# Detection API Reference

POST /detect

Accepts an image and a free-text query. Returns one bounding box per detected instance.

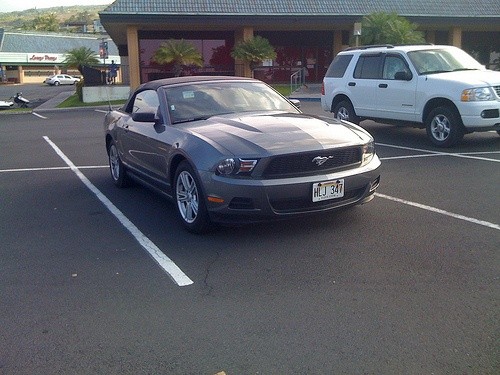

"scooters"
[0,91,34,110]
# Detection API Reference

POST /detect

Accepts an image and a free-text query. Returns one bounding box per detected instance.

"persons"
[107,60,119,85]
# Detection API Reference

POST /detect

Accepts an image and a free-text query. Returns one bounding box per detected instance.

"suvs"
[320,43,500,148]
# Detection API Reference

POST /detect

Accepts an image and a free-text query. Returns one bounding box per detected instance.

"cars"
[45,73,81,87]
[103,76,382,235]
